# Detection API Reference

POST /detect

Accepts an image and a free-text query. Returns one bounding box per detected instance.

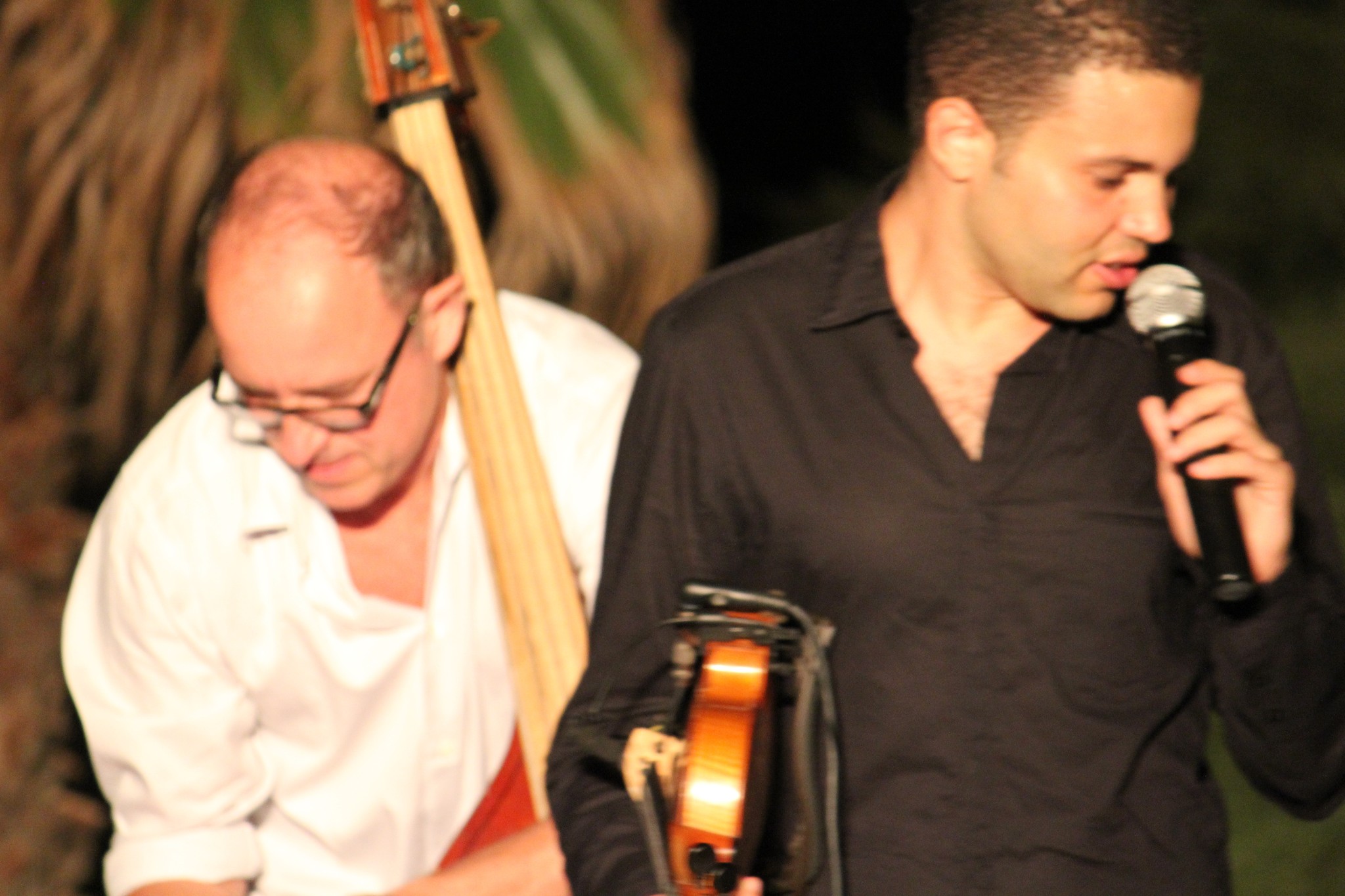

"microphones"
[1123,262,1253,604]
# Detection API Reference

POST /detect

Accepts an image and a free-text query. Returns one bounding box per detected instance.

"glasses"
[210,301,416,434]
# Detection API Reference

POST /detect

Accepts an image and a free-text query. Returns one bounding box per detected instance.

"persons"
[57,134,649,896]
[531,0,1345,896]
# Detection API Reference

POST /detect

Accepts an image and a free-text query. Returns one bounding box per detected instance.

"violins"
[617,580,789,896]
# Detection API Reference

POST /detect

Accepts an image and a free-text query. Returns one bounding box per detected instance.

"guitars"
[348,0,593,879]
